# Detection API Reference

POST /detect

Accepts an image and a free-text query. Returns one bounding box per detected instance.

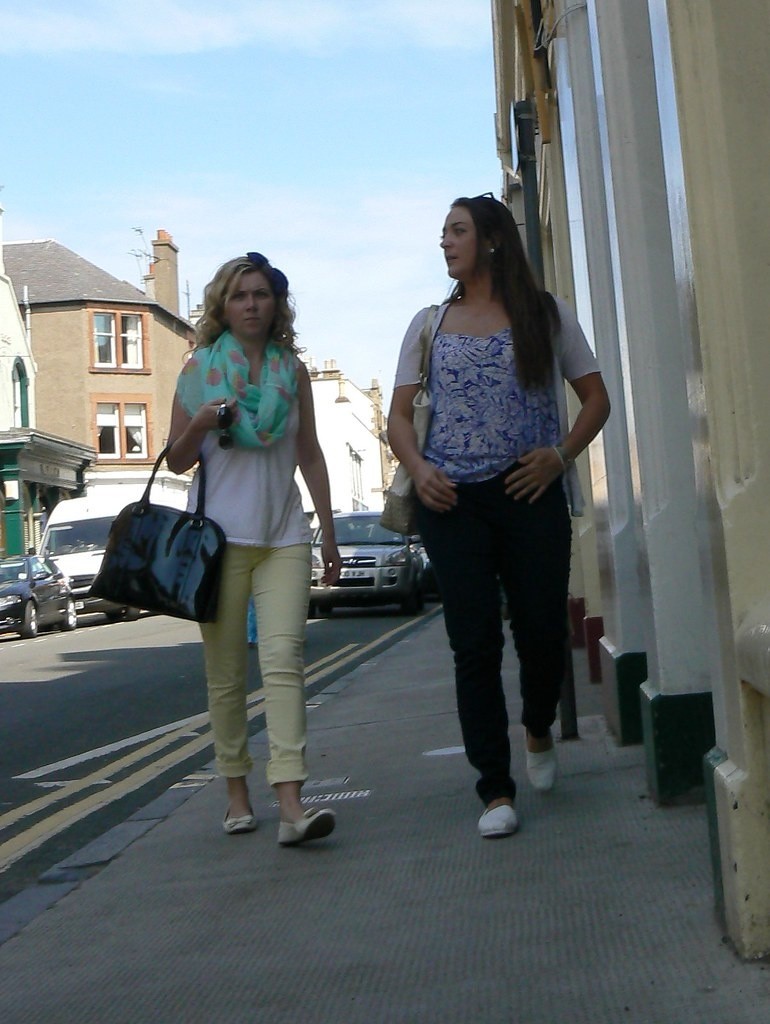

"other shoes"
[222,804,257,833]
[478,804,518,837]
[277,807,335,844]
[525,727,558,791]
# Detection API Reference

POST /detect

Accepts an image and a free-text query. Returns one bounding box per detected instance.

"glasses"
[217,404,234,451]
[473,192,497,204]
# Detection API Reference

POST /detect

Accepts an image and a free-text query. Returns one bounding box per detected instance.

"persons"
[164,251,342,841]
[388,192,612,837]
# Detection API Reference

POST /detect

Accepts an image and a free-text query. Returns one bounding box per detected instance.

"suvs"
[308,510,425,619]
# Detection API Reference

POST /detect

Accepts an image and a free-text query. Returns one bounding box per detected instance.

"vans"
[29,490,189,623]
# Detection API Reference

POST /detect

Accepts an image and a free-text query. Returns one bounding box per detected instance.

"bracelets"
[554,446,574,471]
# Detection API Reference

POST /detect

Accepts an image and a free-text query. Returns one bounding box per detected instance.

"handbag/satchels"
[87,445,226,624]
[379,303,451,536]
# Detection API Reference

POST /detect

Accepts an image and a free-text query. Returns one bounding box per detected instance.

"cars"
[415,540,440,598]
[1,555,77,638]
